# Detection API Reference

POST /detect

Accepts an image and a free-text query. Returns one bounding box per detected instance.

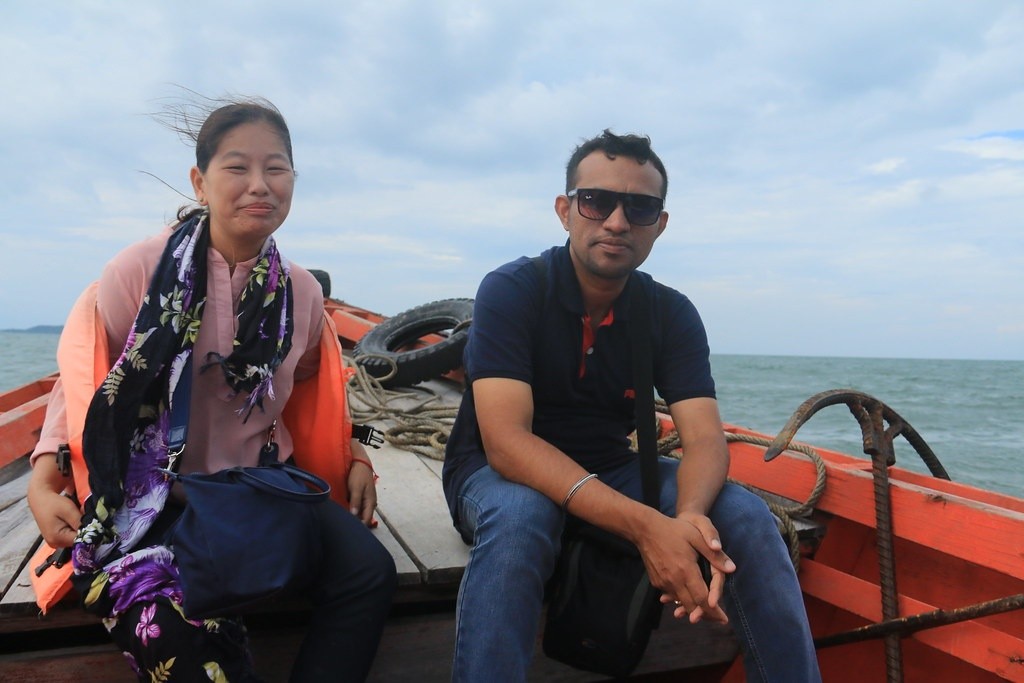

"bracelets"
[562,472,599,512]
[350,457,379,484]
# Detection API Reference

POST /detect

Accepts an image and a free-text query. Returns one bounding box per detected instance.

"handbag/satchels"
[543,533,663,678]
[150,460,398,625]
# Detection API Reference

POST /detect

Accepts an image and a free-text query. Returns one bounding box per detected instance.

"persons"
[29,98,397,683]
[439,132,820,683]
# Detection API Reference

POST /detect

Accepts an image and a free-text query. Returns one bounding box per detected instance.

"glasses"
[567,188,667,226]
